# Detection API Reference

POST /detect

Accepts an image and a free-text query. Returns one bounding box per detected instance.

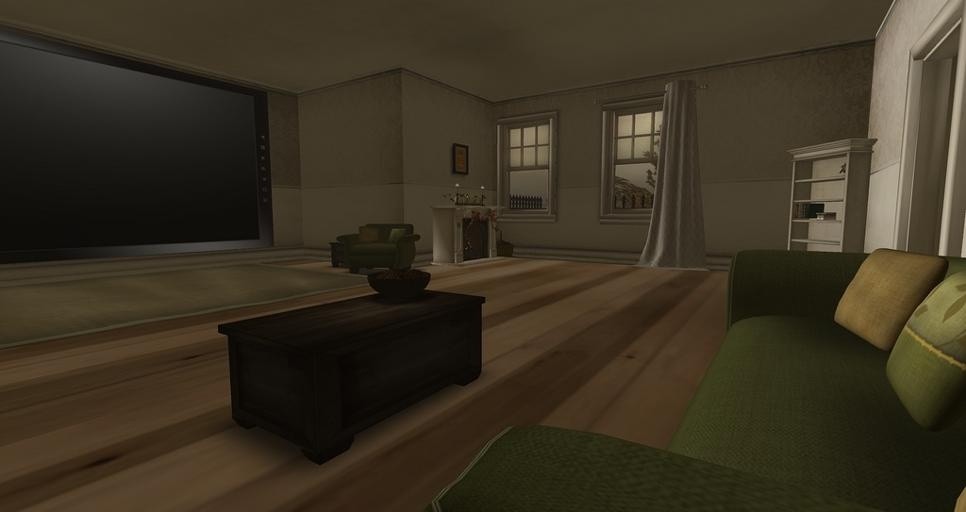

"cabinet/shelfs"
[785,137,875,255]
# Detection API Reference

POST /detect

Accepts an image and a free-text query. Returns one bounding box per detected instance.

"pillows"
[833,243,966,429]
[386,228,407,242]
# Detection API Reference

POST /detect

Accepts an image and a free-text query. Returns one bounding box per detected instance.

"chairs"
[337,222,419,272]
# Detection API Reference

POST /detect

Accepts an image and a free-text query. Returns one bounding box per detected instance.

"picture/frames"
[452,143,469,176]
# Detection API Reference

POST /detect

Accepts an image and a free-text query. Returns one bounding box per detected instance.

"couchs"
[428,250,966,512]
